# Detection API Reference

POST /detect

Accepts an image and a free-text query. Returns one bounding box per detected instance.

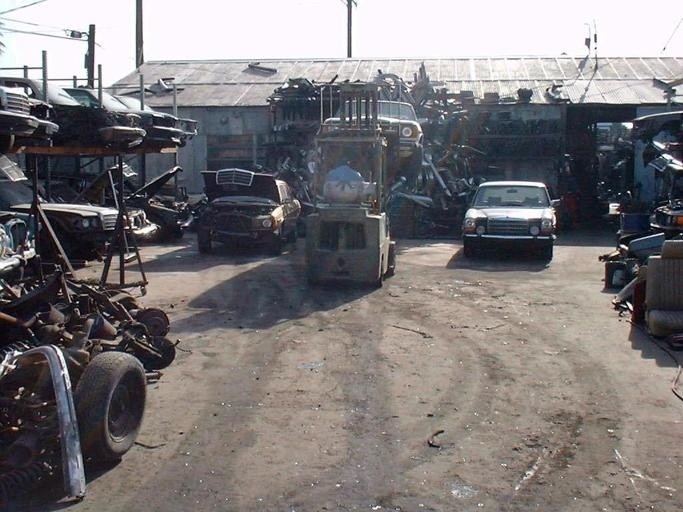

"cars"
[0,75,197,251]
[317,98,423,177]
[196,167,302,254]
[459,179,560,261]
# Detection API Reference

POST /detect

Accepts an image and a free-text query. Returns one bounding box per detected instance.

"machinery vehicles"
[302,129,395,290]
